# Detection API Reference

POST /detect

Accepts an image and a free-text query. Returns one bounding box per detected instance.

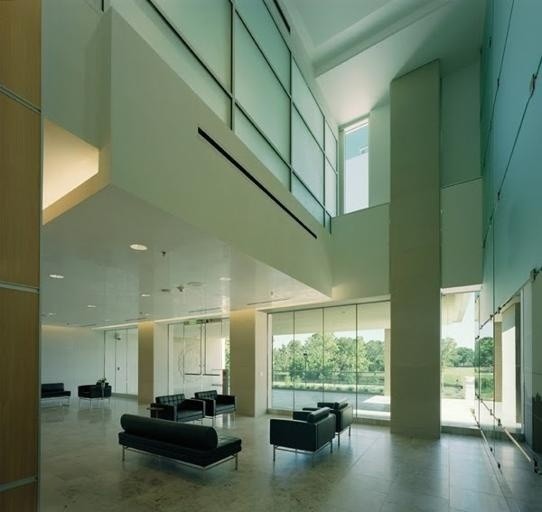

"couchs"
[40,383,71,405]
[118,413,243,481]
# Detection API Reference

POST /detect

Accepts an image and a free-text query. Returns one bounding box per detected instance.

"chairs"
[78,384,111,408]
[271,399,353,460]
[150,389,238,424]
